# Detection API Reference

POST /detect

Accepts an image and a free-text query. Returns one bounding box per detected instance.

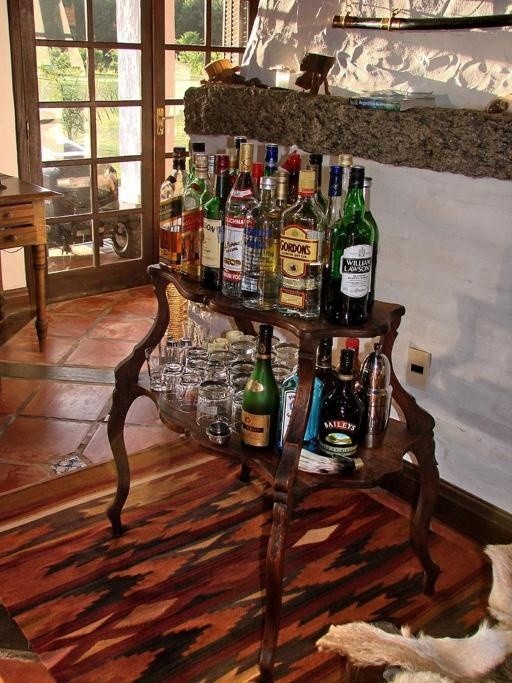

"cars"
[42,132,120,240]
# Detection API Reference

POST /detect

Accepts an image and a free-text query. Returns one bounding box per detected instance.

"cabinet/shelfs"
[100,263,446,673]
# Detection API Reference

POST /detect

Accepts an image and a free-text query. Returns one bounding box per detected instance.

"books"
[348,90,435,111]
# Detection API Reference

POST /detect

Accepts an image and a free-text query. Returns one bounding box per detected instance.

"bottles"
[357,338,393,448]
[155,137,378,326]
[278,333,365,457]
[239,326,277,448]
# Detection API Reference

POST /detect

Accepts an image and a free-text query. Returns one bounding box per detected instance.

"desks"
[0,171,66,354]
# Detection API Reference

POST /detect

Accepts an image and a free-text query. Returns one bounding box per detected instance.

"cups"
[144,331,297,435]
[276,66,291,88]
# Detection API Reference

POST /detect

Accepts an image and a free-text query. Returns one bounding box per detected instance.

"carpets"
[0,424,512,683]
